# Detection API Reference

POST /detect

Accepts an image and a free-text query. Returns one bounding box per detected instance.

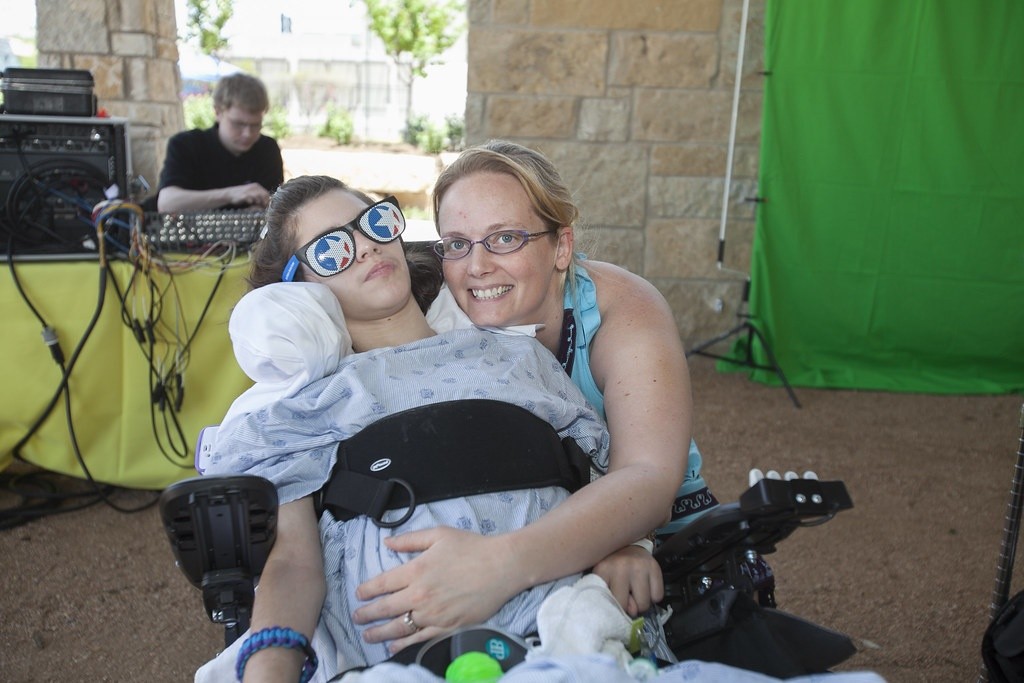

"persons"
[237,173,666,683]
[352,137,778,655]
[142,73,285,214]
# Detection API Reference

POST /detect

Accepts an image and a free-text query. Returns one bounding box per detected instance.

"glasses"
[433,229,558,259]
[294,196,406,277]
[222,113,263,133]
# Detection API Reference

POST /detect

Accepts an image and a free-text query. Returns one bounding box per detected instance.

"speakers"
[0,114,132,257]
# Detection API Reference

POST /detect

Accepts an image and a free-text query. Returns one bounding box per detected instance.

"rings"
[404,611,422,632]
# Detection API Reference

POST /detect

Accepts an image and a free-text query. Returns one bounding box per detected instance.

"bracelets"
[632,538,654,554]
[236,627,319,682]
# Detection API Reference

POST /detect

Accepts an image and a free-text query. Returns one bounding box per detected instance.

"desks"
[0,255,255,490]
